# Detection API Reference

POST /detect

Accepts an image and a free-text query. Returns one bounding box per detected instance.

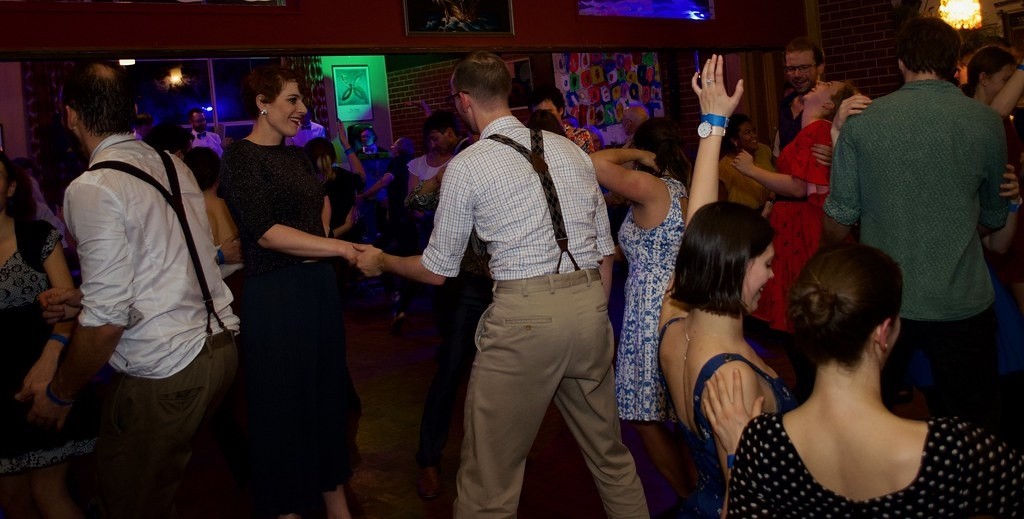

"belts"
[197,331,235,357]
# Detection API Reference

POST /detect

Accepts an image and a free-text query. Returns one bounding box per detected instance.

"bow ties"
[197,132,206,140]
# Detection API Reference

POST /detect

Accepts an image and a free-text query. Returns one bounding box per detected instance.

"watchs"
[698,122,726,139]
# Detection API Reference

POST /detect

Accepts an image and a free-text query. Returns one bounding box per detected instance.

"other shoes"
[389,312,405,334]
[677,495,693,519]
[417,471,439,497]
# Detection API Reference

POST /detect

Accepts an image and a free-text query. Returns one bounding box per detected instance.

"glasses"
[445,91,469,107]
[192,118,206,123]
[784,63,816,74]
[391,145,400,149]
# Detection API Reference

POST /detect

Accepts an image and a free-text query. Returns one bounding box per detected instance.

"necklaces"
[684,316,742,360]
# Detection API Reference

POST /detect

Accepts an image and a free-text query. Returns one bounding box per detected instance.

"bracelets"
[727,454,738,468]
[345,148,355,155]
[701,114,729,127]
[436,175,440,184]
[50,334,67,345]
[1008,204,1019,212]
[47,383,75,406]
[217,249,225,264]
[1016,64,1024,71]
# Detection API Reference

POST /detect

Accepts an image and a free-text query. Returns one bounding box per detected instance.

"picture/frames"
[332,65,373,122]
[575,0,715,23]
[402,0,516,38]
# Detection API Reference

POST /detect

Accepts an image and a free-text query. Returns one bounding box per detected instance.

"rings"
[706,79,713,83]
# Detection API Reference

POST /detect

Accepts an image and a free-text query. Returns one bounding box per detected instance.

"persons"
[0,36,1024,519]
